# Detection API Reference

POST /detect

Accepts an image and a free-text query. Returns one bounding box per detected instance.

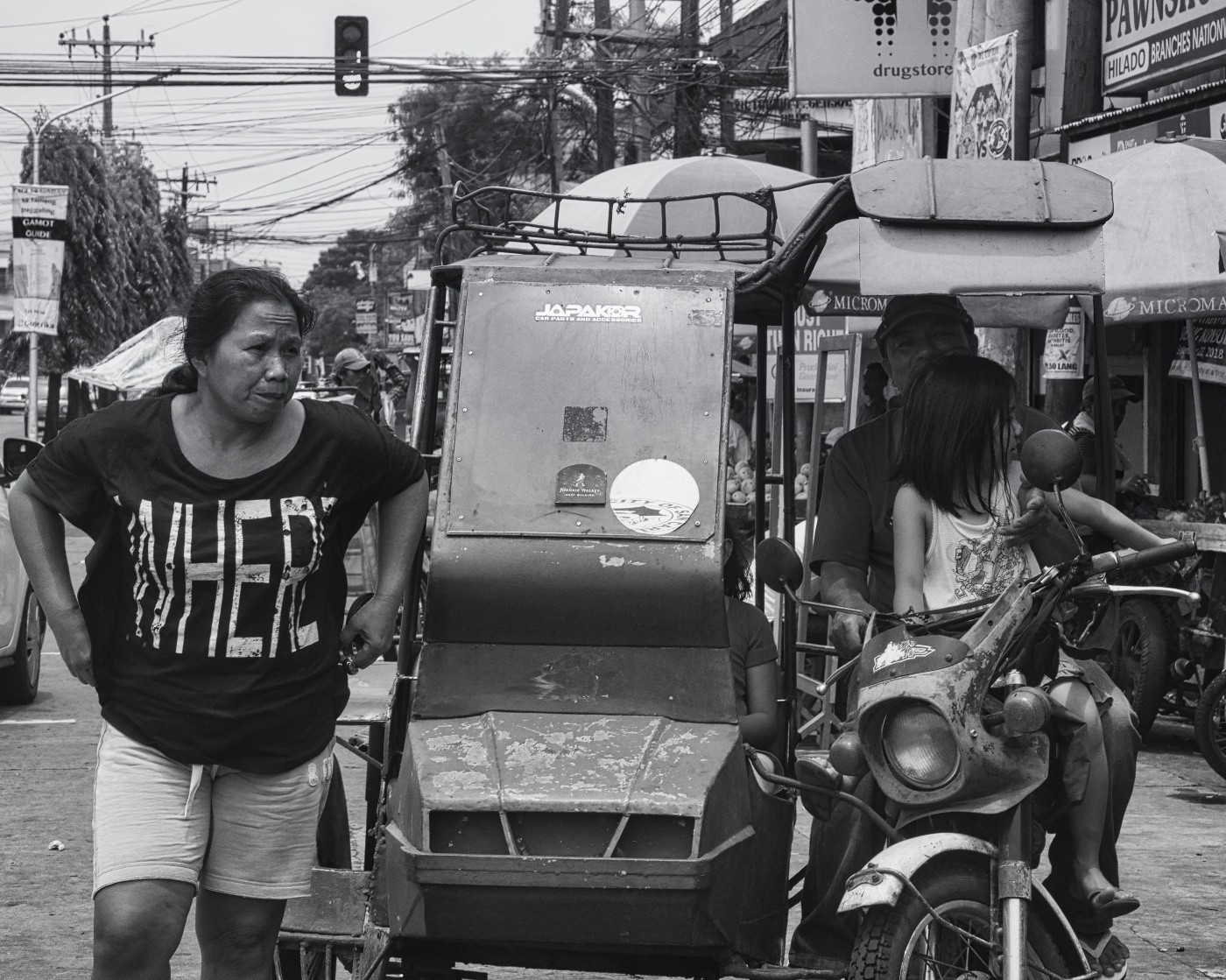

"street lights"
[0,63,187,442]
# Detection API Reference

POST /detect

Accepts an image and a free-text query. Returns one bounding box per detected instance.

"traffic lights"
[333,14,370,97]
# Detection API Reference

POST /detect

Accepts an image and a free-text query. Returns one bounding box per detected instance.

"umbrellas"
[955,131,1226,496]
[508,147,888,612]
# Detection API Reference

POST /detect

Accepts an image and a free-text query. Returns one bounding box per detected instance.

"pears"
[724,461,810,504]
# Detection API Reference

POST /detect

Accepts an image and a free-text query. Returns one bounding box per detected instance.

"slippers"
[1060,932,1129,980]
[795,756,843,821]
[1070,889,1140,934]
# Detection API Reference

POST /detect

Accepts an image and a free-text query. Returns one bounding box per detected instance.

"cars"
[0,433,48,706]
[0,369,98,438]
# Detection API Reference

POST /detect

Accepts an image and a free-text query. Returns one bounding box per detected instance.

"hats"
[873,295,975,359]
[334,348,371,370]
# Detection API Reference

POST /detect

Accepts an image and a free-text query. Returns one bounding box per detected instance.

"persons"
[894,358,1179,920]
[789,295,1140,980]
[856,362,889,426]
[6,267,430,980]
[331,347,408,404]
[1061,375,1142,545]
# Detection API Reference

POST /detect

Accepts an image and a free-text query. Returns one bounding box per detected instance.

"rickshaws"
[271,152,1200,980]
[1054,516,1226,782]
